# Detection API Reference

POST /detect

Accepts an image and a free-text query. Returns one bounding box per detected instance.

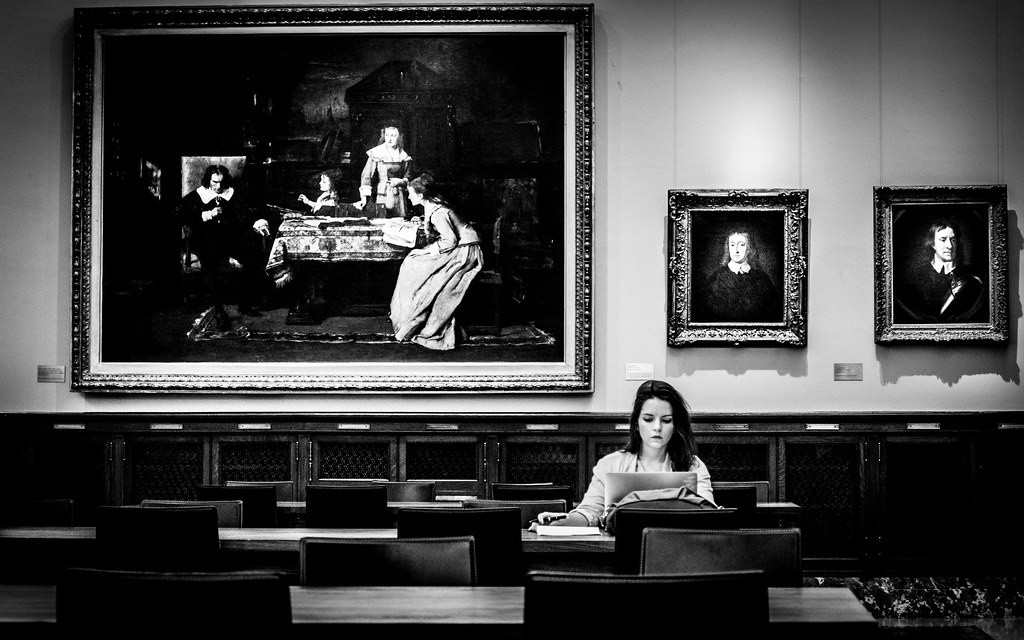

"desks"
[0,584,879,640]
[1,524,616,581]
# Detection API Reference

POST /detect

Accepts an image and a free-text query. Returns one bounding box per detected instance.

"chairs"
[56,479,803,639]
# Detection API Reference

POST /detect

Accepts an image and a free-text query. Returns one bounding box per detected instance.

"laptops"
[603,472,697,512]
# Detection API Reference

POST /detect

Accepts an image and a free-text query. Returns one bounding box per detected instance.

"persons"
[528,380,713,532]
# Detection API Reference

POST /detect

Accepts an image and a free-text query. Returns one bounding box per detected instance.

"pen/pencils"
[529,516,566,524]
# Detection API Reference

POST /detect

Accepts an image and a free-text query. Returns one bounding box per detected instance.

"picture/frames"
[873,185,1010,344]
[666,189,809,347]
[69,4,596,395]
